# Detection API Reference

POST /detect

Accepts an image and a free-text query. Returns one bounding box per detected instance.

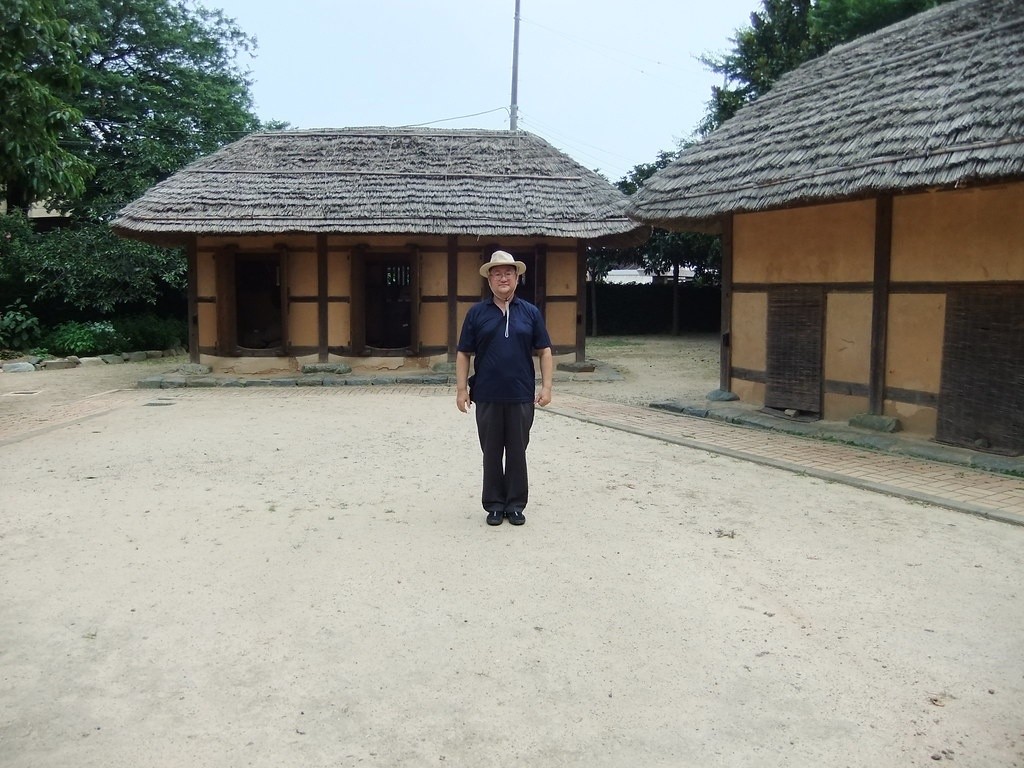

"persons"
[456,250,552,526]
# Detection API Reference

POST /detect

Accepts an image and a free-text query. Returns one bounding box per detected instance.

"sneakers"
[506,510,525,525]
[486,509,505,526]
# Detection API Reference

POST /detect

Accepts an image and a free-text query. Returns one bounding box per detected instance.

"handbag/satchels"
[468,373,479,402]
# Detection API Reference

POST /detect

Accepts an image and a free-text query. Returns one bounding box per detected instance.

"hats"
[479,250,526,277]
